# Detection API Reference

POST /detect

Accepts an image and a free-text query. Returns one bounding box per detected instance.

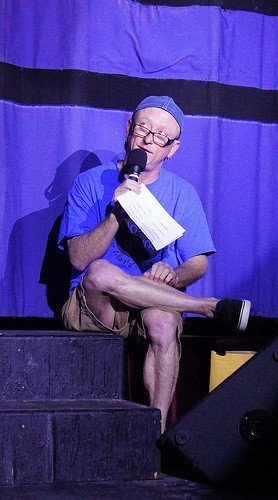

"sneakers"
[209,298,251,331]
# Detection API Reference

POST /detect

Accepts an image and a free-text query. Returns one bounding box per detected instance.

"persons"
[56,96,252,433]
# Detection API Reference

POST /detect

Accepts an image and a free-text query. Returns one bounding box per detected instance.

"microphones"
[127,149,147,183]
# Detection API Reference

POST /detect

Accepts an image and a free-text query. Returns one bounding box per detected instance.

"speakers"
[160,336,278,494]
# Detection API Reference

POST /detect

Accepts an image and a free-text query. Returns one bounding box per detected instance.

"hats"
[132,96,185,135]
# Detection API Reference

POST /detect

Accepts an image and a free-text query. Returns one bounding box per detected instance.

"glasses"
[131,122,176,149]
